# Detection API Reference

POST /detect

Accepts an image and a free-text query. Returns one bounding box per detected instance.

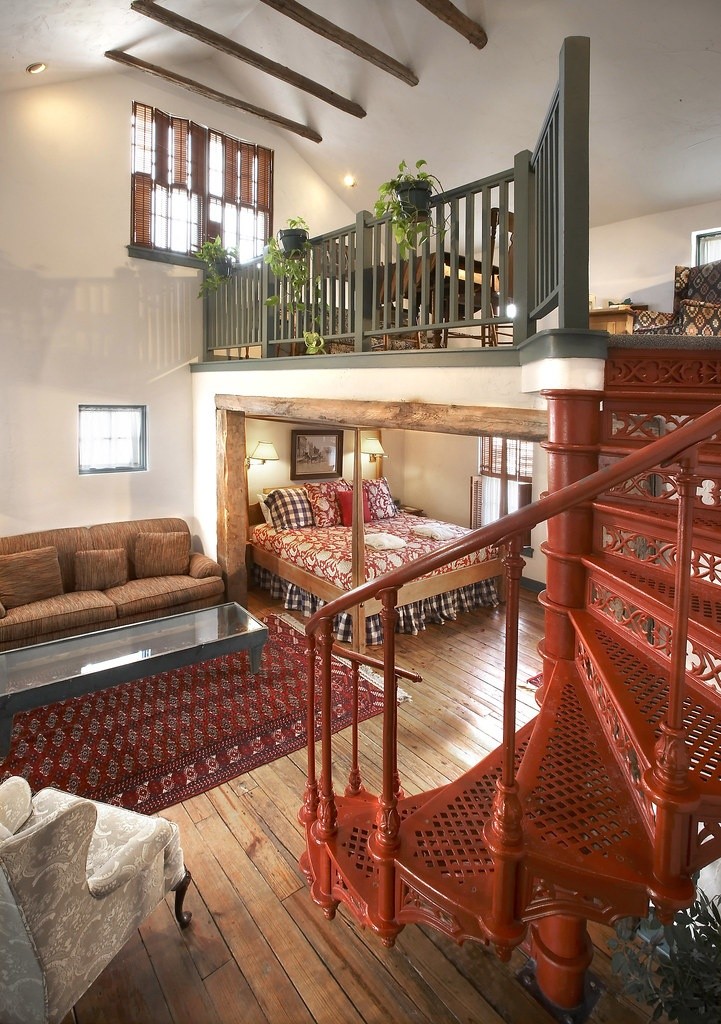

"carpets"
[0,613,412,816]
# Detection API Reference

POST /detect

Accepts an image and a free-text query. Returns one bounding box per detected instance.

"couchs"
[277,302,444,355]
[634,259,721,336]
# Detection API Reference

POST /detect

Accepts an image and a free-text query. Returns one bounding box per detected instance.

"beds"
[248,503,501,645]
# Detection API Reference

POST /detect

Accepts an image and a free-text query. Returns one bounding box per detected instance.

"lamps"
[194,233,239,297]
[244,441,279,470]
[361,437,389,462]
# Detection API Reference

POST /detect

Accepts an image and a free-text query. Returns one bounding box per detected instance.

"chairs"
[443,207,514,348]
[0,775,192,1023]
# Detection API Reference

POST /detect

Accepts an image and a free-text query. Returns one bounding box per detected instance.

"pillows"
[74,548,127,591]
[135,531,190,578]
[0,546,64,608]
[411,525,457,541]
[364,533,406,550]
[256,476,402,533]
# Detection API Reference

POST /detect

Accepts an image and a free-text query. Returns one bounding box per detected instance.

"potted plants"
[262,216,330,323]
[372,160,453,261]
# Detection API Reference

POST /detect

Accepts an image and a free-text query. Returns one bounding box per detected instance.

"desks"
[588,307,635,335]
[379,251,499,349]
[0,601,268,755]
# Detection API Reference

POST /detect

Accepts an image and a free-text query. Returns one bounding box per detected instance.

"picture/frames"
[290,430,344,480]
